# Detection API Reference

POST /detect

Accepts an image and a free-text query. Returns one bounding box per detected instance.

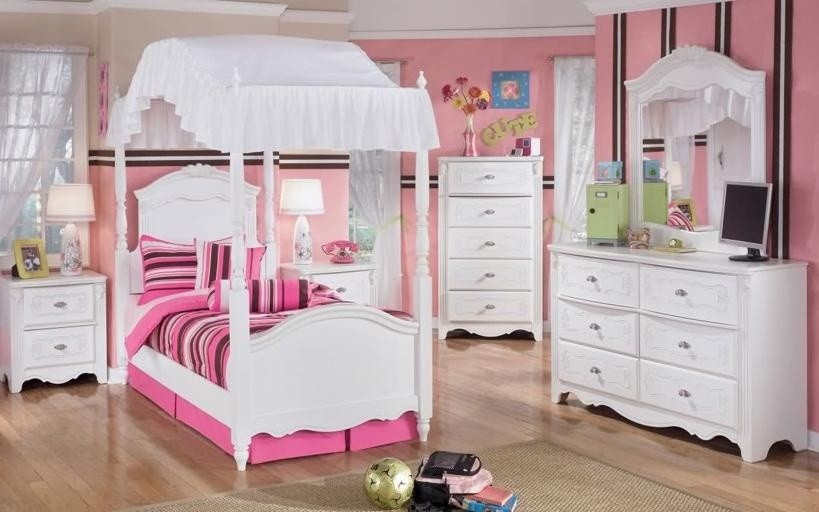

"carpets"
[98,442,740,512]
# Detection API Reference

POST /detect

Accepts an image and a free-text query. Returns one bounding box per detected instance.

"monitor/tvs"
[718,181,773,261]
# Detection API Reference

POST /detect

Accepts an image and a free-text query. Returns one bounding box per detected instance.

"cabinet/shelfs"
[547,238,808,465]
[441,160,542,340]
[0,265,108,391]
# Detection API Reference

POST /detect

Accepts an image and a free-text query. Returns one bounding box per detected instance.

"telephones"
[321,241,357,263]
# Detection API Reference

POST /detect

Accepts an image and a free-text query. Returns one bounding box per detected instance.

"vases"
[463,114,479,157]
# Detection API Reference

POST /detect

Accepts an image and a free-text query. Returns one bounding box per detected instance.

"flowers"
[442,76,490,117]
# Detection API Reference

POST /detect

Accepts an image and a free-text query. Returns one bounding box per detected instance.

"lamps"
[279,177,328,265]
[47,182,97,274]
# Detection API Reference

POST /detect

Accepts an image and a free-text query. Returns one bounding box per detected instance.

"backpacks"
[413,450,493,510]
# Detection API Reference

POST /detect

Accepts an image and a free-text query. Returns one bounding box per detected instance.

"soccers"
[363,457,415,510]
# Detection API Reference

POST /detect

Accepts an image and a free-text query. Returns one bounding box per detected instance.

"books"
[464,485,513,506]
[462,492,517,511]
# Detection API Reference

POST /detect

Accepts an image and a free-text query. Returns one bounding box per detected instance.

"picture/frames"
[14,238,45,279]
[489,69,530,108]
[672,196,697,227]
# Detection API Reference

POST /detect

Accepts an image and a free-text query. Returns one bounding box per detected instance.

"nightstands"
[280,252,377,307]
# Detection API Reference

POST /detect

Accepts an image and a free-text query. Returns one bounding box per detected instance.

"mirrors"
[625,45,766,253]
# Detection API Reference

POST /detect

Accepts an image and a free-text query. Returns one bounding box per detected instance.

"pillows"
[137,237,203,302]
[198,241,266,306]
[204,276,312,314]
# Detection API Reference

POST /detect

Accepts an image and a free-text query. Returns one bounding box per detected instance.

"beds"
[119,161,430,463]
